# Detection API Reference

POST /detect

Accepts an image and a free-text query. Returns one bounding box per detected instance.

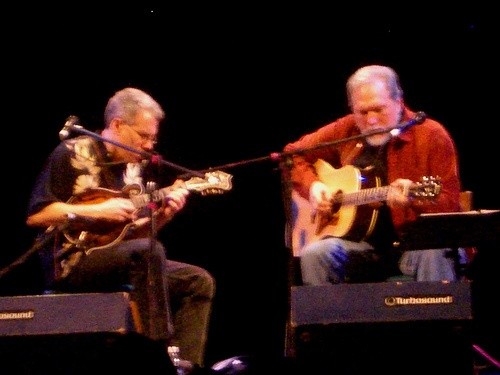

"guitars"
[62,170,233,255]
[291,157,442,257]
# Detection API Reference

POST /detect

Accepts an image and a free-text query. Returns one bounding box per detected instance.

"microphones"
[390,112,426,137]
[45,215,75,235]
[59,116,78,139]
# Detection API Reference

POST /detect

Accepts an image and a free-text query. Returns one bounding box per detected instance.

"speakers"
[0,292,178,375]
[285,280,473,375]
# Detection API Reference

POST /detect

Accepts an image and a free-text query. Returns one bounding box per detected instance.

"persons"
[26,88,215,375]
[278,65,460,286]
